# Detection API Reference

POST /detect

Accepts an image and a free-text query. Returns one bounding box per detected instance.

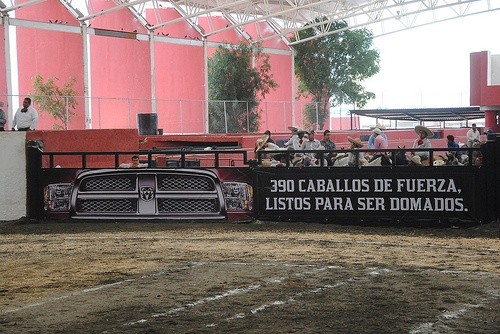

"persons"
[447,135,462,157]
[0,102,7,132]
[252,126,339,167]
[11,98,38,131]
[466,122,480,148]
[410,125,435,161]
[129,156,143,169]
[367,124,388,162]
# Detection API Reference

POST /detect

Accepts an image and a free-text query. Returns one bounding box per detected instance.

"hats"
[346,136,364,147]
[288,126,300,132]
[293,128,310,135]
[371,123,386,130]
[414,125,434,137]
[255,135,269,152]
[371,127,381,134]
